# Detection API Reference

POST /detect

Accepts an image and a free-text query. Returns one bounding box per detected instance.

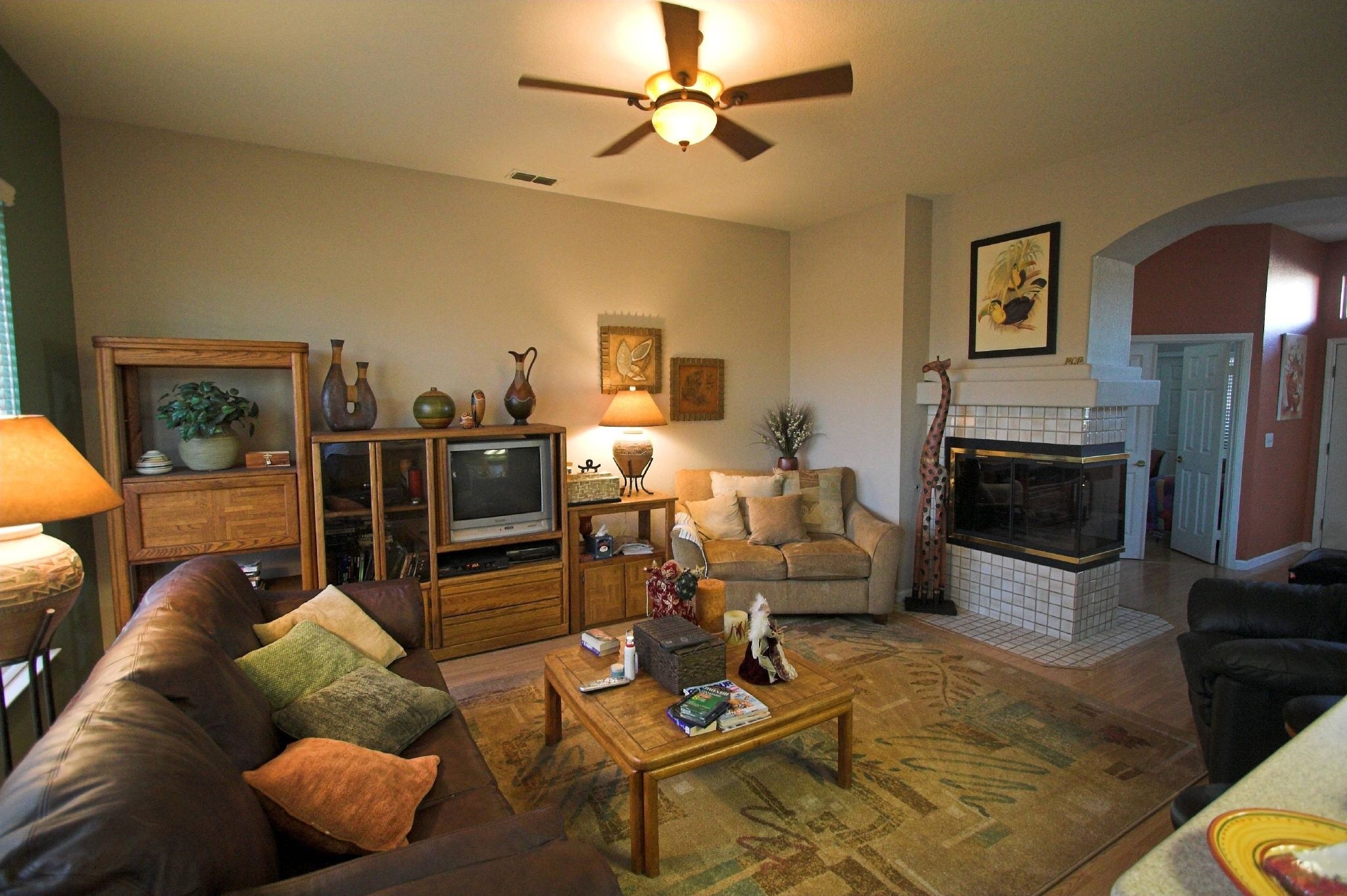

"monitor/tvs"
[448,439,554,543]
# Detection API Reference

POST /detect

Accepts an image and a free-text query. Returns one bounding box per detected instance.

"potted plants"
[154,375,260,471]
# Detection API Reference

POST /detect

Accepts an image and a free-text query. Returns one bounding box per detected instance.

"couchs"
[1175,565,1347,782]
[0,550,624,896]
[669,466,906,622]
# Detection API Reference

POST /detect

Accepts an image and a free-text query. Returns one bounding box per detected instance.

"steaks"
[1263,852,1347,896]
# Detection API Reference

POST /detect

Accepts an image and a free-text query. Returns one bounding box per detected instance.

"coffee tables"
[538,622,861,871]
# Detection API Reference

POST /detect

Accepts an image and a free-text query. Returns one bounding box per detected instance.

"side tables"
[569,488,681,631]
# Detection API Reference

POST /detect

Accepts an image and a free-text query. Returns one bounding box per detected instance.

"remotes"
[579,676,631,692]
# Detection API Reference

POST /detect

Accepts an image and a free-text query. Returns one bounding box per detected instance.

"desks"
[1103,692,1347,896]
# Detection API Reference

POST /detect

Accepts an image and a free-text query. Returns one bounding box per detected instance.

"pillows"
[743,492,811,545]
[244,737,443,855]
[252,588,408,668]
[709,470,784,533]
[234,616,369,704]
[266,668,461,752]
[683,490,749,542]
[773,465,848,537]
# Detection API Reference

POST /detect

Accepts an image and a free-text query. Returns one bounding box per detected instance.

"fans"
[511,0,856,166]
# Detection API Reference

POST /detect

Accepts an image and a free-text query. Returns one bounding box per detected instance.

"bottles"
[413,387,456,429]
[504,347,537,426]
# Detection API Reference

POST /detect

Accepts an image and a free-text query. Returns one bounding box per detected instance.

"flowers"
[744,395,828,458]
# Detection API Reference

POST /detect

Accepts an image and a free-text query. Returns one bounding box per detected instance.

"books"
[666,679,772,737]
[581,629,621,657]
[236,561,266,590]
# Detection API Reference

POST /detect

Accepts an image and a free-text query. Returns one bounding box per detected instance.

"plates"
[1206,808,1347,896]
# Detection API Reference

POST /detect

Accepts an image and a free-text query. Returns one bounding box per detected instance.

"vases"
[776,457,799,471]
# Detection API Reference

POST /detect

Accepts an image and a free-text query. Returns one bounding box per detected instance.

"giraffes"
[912,355,952,611]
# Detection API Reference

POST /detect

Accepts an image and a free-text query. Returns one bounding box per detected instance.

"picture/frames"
[966,220,1063,360]
[1276,334,1308,422]
[669,355,725,421]
[597,325,664,395]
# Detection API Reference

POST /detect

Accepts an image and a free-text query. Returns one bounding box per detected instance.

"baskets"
[567,471,620,504]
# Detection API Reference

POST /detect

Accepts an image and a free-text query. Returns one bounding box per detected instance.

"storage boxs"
[633,614,727,695]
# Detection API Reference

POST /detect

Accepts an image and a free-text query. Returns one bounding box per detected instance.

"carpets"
[463,607,1206,896]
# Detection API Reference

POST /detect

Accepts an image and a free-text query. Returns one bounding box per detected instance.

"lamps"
[597,389,667,499]
[643,68,723,154]
[0,412,128,658]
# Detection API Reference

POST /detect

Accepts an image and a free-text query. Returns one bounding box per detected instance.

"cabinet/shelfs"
[85,332,315,632]
[309,422,569,658]
